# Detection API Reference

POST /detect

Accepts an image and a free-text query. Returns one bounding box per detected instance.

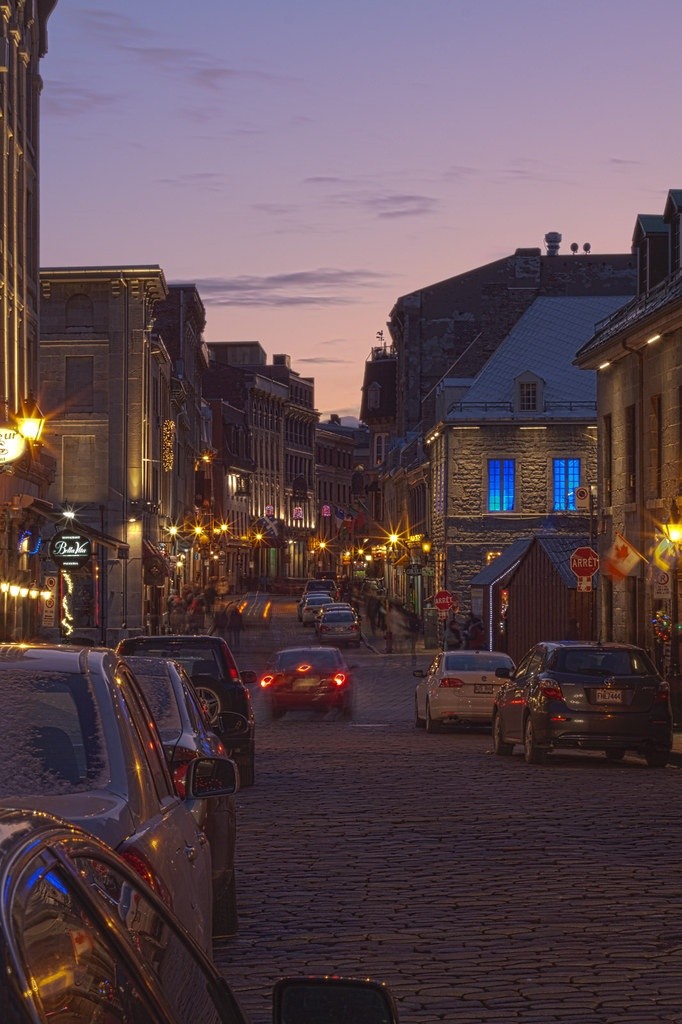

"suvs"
[118,633,256,789]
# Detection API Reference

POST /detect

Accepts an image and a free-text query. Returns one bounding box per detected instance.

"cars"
[116,654,250,945]
[0,642,239,969]
[258,646,359,721]
[295,577,363,645]
[0,804,402,1024]
[413,650,518,734]
[491,640,675,767]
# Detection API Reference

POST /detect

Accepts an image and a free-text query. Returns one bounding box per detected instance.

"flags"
[334,507,345,529]
[603,534,641,583]
[343,513,355,529]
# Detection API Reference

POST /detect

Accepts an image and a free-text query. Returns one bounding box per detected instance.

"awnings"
[20,494,130,561]
[141,538,170,576]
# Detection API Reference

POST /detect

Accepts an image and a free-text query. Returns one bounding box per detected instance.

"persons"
[167,569,236,635]
[213,604,246,648]
[336,574,421,667]
[445,611,484,654]
